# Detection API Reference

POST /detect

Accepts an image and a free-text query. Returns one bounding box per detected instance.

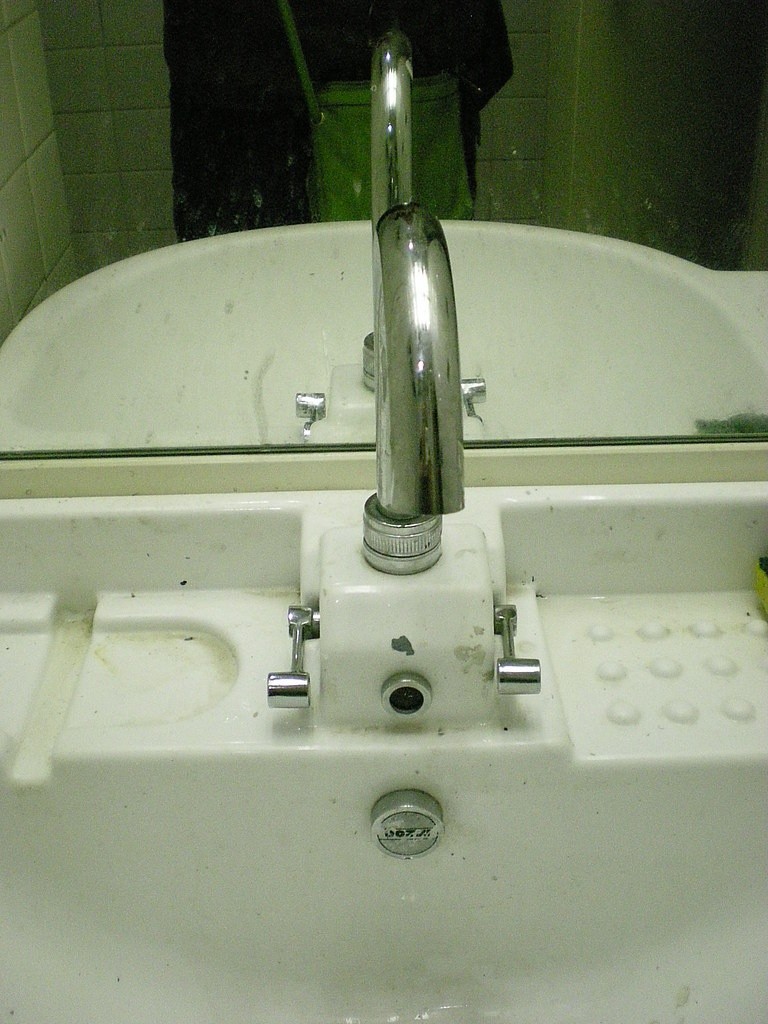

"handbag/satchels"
[310,71,474,223]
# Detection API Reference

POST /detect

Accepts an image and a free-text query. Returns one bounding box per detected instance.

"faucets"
[361,25,414,394]
[363,198,468,576]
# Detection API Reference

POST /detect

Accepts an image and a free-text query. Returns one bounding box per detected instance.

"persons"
[162,0,514,243]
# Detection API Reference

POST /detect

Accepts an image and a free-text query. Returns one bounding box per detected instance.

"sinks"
[0,759,768,1024]
[1,227,766,427]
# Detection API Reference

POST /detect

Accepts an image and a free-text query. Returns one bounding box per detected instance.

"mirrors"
[2,2,767,457]
